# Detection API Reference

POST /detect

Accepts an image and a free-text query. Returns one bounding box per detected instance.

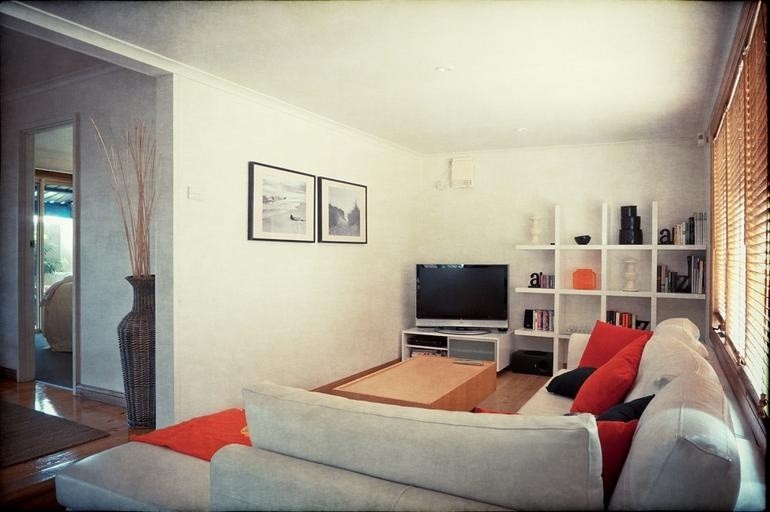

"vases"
[117,276,155,427]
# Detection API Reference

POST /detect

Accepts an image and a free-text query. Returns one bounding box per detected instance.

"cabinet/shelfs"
[514,201,706,376]
[402,327,513,373]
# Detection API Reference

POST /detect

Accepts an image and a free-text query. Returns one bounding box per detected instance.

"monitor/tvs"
[415,264,508,335]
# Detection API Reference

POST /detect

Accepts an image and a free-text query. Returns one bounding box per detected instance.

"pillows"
[242,381,606,512]
[470,320,655,507]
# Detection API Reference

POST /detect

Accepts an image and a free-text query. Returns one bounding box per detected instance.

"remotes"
[454,358,483,366]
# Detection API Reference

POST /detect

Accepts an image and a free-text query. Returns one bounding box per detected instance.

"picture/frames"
[318,176,367,244]
[248,162,316,242]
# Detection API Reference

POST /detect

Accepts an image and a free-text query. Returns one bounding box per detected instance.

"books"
[537,275,555,289]
[607,311,650,332]
[533,308,553,331]
[674,211,708,244]
[657,254,706,295]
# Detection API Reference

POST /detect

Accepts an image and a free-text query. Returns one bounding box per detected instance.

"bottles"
[529,215,541,246]
[572,268,596,290]
[622,259,640,291]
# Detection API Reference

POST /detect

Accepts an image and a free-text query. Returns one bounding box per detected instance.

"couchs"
[52,316,741,511]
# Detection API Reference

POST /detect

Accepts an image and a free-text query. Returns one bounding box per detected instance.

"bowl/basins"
[575,236,591,244]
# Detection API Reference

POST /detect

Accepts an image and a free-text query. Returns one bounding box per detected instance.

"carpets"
[0,400,110,469]
